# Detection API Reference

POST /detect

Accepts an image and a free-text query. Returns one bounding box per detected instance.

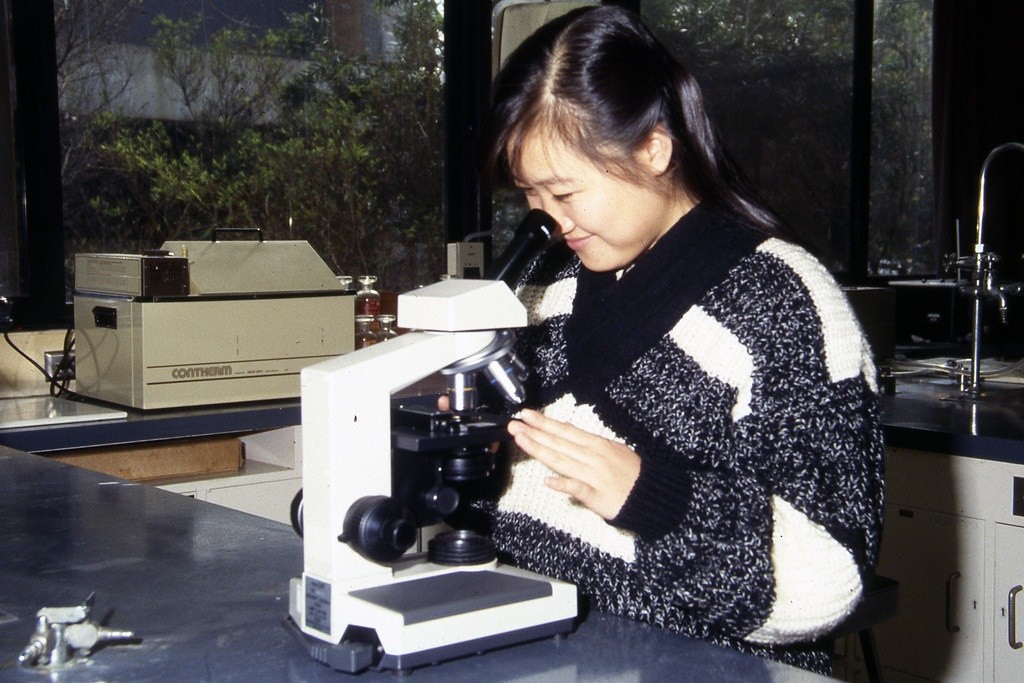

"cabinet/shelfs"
[825,356,1024,683]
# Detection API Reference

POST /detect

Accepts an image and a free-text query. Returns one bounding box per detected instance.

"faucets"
[947,143,1023,398]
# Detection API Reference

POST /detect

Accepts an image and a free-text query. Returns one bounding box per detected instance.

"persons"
[439,5,886,674]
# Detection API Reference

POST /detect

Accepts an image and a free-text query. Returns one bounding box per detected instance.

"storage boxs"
[44,435,244,484]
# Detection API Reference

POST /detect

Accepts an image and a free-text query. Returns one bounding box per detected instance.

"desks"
[0,392,843,683]
